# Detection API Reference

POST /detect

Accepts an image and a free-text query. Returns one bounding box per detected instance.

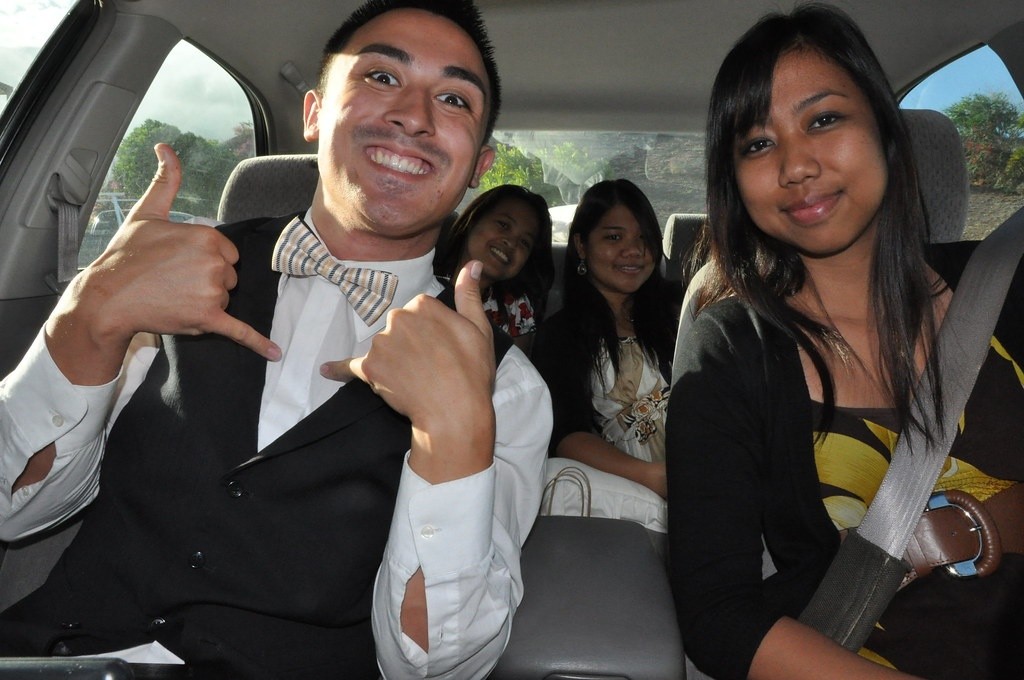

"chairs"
[672,110,973,373]
[216,154,319,224]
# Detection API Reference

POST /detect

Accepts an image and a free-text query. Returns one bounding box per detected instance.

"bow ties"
[271,216,398,327]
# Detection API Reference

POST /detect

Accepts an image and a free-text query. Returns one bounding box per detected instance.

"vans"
[88,208,194,258]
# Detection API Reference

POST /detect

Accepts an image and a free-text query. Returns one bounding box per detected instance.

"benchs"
[443,208,707,322]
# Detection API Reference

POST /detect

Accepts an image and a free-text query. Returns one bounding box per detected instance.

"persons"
[431,180,678,535]
[0,0,555,680]
[664,0,1024,680]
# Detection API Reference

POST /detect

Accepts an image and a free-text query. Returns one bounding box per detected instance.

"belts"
[839,482,1024,579]
[128,664,225,680]
